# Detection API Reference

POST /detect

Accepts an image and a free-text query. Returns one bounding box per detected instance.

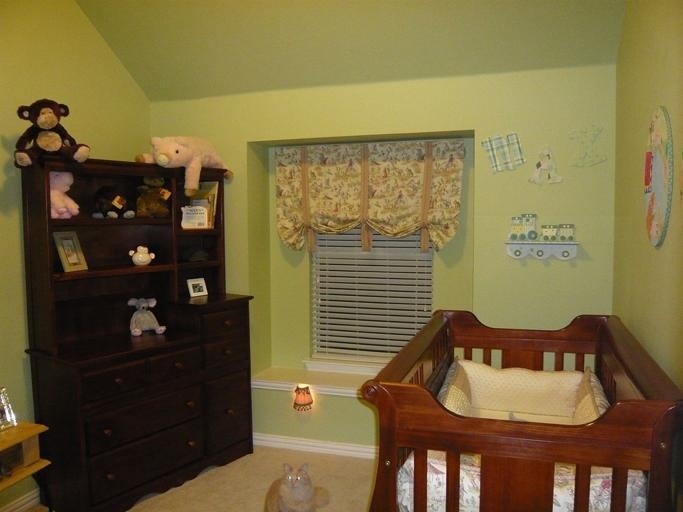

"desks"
[0,421,53,493]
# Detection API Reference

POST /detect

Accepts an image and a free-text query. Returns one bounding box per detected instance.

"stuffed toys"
[136,177,171,218]
[135,135,234,197]
[127,297,167,336]
[12,99,90,168]
[88,187,135,219]
[50,171,80,219]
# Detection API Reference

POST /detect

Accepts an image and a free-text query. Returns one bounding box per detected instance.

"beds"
[360,310,683,512]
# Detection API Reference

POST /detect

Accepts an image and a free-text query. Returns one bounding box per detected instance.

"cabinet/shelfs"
[21,153,253,512]
